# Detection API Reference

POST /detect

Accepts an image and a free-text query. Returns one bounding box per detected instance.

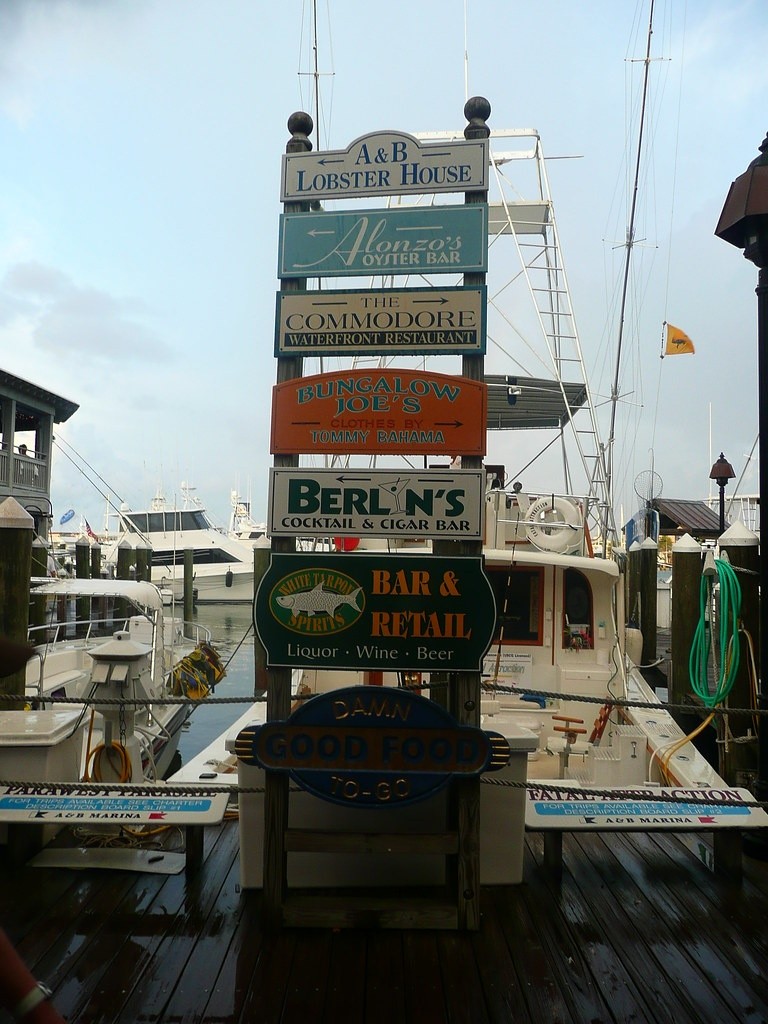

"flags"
[666,325,694,356]
[85,520,98,541]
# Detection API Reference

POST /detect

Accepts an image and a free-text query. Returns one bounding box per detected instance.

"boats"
[160,129,731,793]
[0,579,211,847]
[46,482,266,577]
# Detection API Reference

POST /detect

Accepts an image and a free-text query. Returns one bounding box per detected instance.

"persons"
[47,554,57,578]
[16,443,38,486]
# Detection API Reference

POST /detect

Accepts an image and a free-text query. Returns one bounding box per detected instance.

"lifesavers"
[526,498,578,549]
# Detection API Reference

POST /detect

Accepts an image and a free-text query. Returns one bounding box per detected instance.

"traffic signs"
[280,134,489,197]
[267,366,486,459]
[277,201,488,278]
[267,464,486,541]
[269,286,490,358]
